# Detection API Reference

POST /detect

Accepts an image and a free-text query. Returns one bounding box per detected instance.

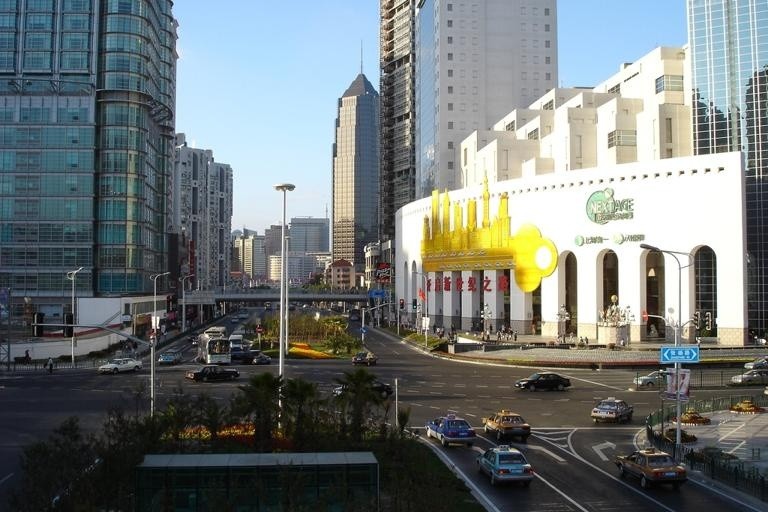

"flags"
[418,287,426,303]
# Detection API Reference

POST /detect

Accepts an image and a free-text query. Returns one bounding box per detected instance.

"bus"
[237,307,248,319]
[197,326,231,364]
[263,302,271,310]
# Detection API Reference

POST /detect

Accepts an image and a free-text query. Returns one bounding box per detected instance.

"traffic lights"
[692,312,700,330]
[399,299,404,309]
[412,299,416,309]
[703,312,711,331]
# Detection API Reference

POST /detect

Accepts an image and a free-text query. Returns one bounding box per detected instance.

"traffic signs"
[660,347,699,365]
[359,328,368,334]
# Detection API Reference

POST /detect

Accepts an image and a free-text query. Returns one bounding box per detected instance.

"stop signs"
[640,311,648,323]
[255,327,262,333]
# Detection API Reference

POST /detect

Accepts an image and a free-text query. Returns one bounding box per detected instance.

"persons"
[620,337,625,346]
[108,325,181,352]
[46,356,53,372]
[24,349,30,365]
[430,323,518,343]
[547,330,589,347]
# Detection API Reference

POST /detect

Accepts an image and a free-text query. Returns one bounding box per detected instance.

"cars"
[424,409,534,487]
[157,350,180,366]
[633,370,666,388]
[97,358,142,375]
[731,355,767,386]
[242,350,271,365]
[331,380,393,401]
[613,450,687,490]
[185,364,239,383]
[514,372,570,392]
[590,397,633,424]
[351,352,376,367]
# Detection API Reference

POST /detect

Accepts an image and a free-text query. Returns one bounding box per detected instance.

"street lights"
[412,270,428,348]
[480,303,491,340]
[275,184,294,432]
[556,304,570,344]
[178,273,195,334]
[638,243,695,468]
[149,271,170,417]
[67,267,84,367]
